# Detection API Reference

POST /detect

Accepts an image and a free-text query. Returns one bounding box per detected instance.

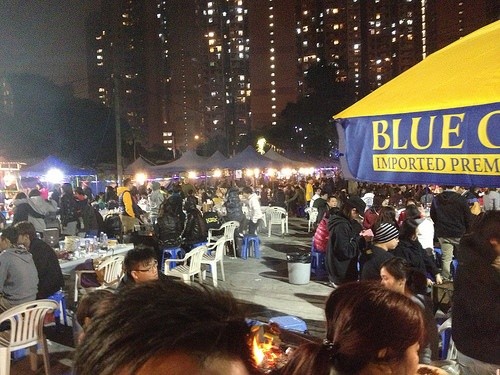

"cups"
[65,236,80,252]
[59,240,65,251]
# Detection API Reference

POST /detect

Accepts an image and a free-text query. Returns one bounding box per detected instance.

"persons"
[0,163,500,375]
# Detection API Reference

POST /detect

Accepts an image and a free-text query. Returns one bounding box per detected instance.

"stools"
[311,250,330,281]
[268,316,307,335]
[242,233,261,259]
[44,287,66,329]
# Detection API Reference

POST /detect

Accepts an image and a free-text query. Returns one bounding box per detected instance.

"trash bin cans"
[285,251,314,285]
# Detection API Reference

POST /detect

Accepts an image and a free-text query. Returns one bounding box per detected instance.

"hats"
[375,223,400,243]
[346,195,366,216]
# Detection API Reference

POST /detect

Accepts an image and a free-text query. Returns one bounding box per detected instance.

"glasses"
[133,260,159,271]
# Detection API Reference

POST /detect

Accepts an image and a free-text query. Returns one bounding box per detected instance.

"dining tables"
[56,242,134,300]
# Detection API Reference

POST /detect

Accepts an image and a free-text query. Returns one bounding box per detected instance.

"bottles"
[203,201,208,212]
[100,232,109,245]
[84,235,99,253]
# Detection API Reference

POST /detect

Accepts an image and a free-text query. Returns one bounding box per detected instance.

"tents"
[328,20,500,189]
[123,145,311,179]
[19,153,99,198]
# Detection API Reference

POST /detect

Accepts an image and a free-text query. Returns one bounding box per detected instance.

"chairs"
[208,220,241,258]
[305,206,319,233]
[163,244,204,286]
[73,255,126,302]
[163,247,186,269]
[0,297,58,375]
[256,204,289,237]
[196,235,227,287]
